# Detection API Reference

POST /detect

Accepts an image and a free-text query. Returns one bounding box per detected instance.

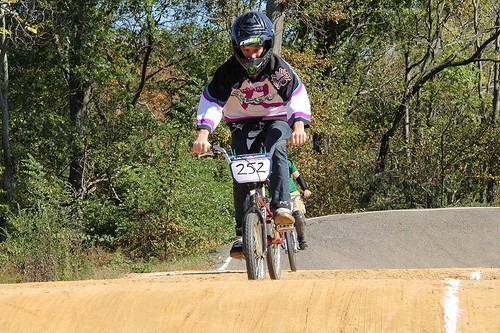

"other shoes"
[299,241,308,250]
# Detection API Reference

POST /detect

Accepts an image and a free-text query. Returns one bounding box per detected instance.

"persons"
[190,11,314,260]
[264,157,311,250]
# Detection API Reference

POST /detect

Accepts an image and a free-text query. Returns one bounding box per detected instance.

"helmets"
[231,12,274,78]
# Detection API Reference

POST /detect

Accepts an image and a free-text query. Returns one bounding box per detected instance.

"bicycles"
[197,137,294,279]
[281,193,304,273]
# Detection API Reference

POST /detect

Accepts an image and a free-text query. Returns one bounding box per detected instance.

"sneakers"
[229,240,245,259]
[273,200,295,232]
